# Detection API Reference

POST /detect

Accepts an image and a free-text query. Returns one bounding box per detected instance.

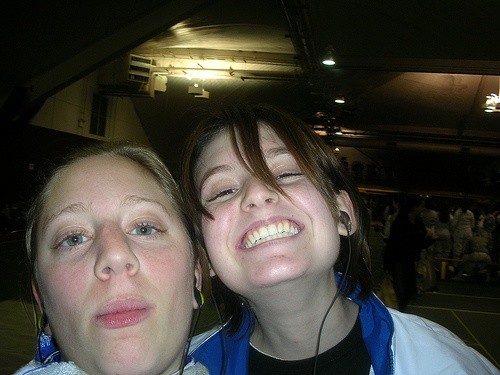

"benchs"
[435,256,500,280]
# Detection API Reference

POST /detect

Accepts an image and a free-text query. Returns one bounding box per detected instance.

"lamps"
[322,48,335,67]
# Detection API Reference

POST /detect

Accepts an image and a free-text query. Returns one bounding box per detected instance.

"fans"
[334,89,346,104]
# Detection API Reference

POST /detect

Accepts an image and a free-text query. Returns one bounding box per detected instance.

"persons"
[174,99,500,375]
[381,194,438,314]
[0,139,211,375]
[364,192,500,278]
